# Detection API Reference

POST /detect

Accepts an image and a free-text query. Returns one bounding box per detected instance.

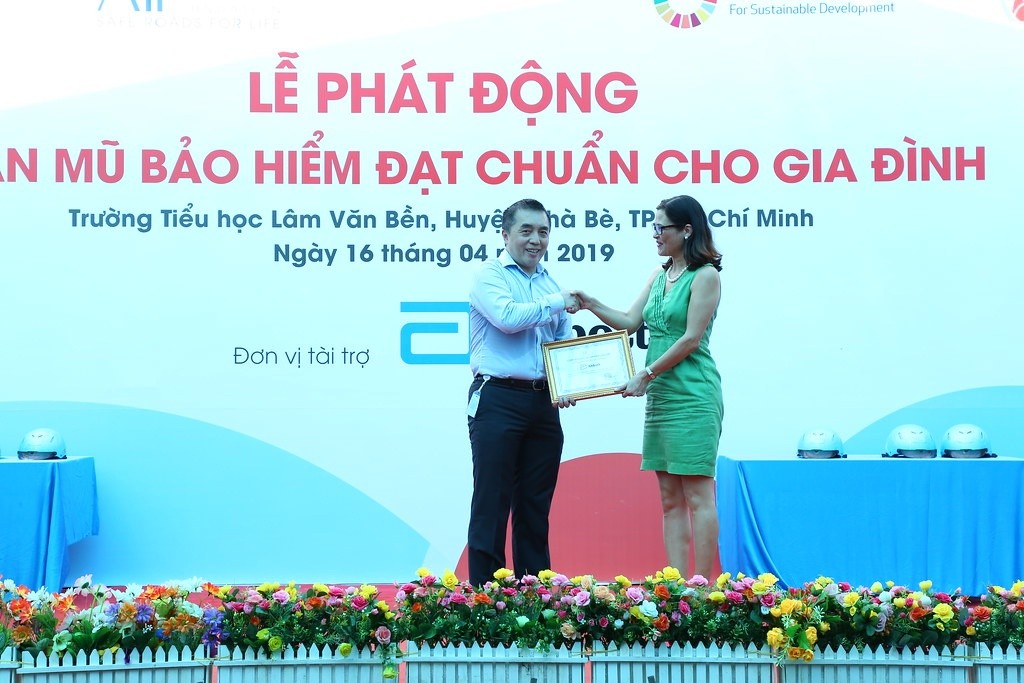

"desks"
[716,455,1024,597]
[0,454,100,603]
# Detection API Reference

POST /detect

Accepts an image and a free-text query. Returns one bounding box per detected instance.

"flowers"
[1,568,1023,678]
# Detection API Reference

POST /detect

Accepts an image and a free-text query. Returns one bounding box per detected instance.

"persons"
[568,194,725,583]
[465,199,572,583]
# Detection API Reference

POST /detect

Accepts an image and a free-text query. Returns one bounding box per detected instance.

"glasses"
[652,223,684,235]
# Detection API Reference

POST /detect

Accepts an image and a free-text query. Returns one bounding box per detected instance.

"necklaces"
[665,264,692,283]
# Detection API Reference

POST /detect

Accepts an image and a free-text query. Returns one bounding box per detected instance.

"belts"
[473,376,549,391]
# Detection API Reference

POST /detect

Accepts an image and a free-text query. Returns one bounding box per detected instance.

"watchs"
[645,365,657,381]
